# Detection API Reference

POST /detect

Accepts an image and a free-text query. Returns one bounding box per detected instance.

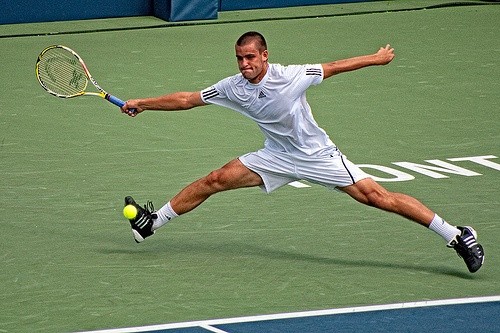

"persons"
[120,31,485,274]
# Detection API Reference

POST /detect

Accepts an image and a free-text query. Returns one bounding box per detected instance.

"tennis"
[123,203,137,220]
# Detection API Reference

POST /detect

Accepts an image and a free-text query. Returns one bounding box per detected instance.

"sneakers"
[123,196,158,242]
[446,225,484,273]
